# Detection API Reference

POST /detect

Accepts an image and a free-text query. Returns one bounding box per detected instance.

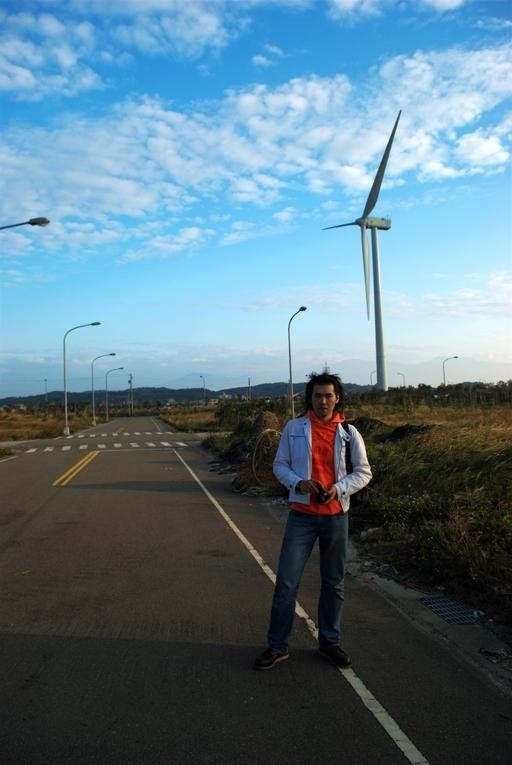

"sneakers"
[253,645,289,670]
[317,639,353,669]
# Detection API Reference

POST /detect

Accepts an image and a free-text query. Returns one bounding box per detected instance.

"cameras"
[315,484,331,504]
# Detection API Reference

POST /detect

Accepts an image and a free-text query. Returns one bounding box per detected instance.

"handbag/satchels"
[341,420,370,511]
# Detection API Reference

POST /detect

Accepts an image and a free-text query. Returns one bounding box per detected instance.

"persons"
[246,370,375,673]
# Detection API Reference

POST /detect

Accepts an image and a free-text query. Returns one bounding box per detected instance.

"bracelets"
[296,480,301,492]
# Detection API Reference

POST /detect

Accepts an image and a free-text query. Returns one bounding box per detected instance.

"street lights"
[91,353,116,427]
[442,355,459,387]
[105,366,124,421]
[200,375,206,406]
[126,374,134,417]
[397,372,405,386]
[0,216,50,230]
[63,322,101,436]
[287,305,307,419]
